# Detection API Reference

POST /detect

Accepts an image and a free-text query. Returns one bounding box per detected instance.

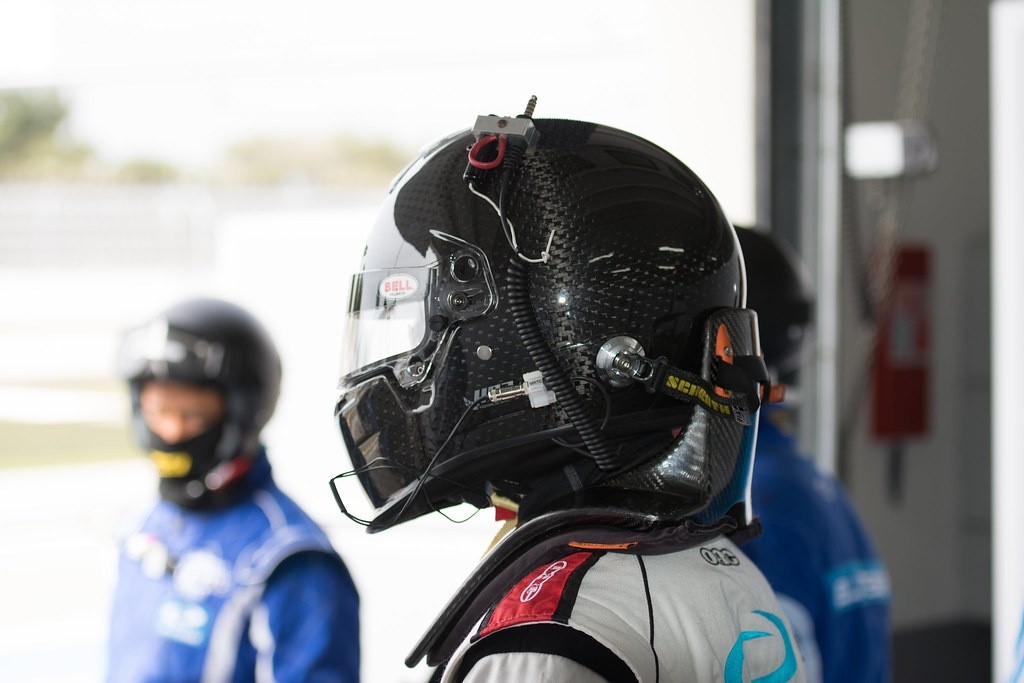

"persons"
[329,93,806,682]
[733,225,894,682]
[106,296,360,683]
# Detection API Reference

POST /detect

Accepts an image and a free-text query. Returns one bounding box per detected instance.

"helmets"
[337,118,750,533]
[130,301,280,507]
[736,227,806,383]
